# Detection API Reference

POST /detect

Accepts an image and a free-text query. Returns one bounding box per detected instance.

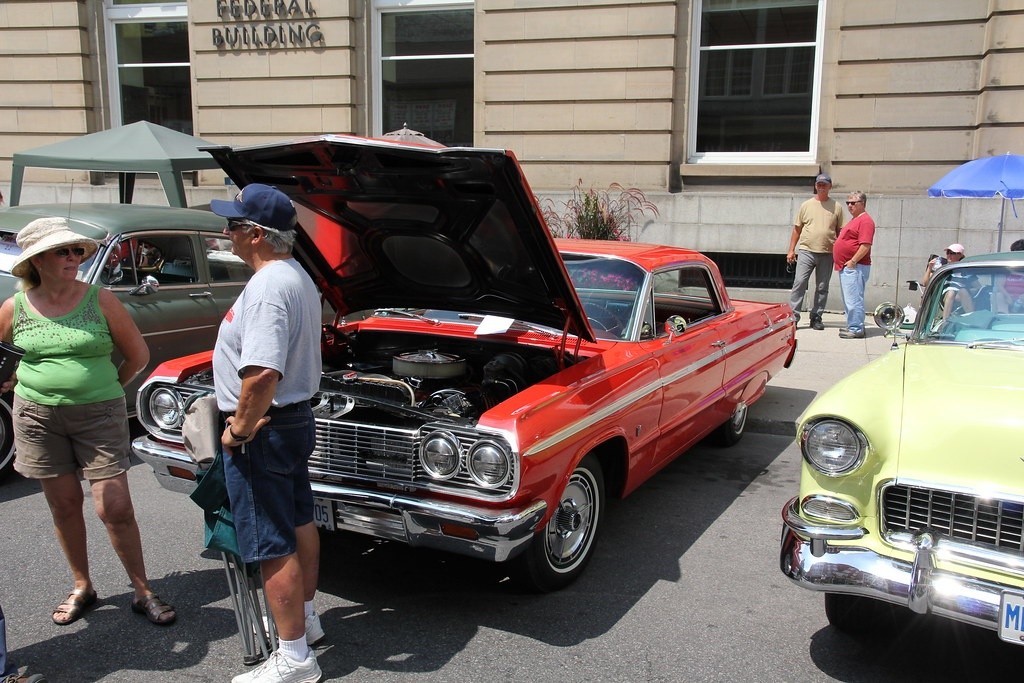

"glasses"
[226,216,279,234]
[43,248,85,257]
[947,250,961,255]
[846,201,862,205]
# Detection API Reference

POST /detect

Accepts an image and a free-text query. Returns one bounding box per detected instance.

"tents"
[10,120,231,208]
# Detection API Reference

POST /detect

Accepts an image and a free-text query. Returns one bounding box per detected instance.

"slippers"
[52,590,97,624]
[132,591,177,625]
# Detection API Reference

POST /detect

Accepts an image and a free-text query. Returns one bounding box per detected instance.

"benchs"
[579,297,713,337]
[154,257,255,284]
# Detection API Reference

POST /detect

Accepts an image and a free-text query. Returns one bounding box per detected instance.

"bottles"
[931,255,942,271]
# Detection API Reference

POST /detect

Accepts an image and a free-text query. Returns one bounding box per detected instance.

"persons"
[0,366,44,683]
[212,185,326,683]
[787,174,847,330]
[833,191,875,338]
[993,239,1024,314]
[0,217,176,624]
[922,244,982,322]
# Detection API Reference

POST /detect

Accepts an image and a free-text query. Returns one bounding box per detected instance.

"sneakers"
[230,645,323,683]
[809,315,825,329]
[252,610,326,645]
[0,673,49,683]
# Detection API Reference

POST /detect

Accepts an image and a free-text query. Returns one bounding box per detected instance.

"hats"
[816,174,831,186]
[11,217,98,278]
[944,243,964,258]
[211,183,298,231]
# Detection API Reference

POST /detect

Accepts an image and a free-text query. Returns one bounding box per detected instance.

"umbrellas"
[927,151,1024,253]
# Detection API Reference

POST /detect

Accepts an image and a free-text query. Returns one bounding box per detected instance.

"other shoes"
[839,327,866,333]
[839,331,864,339]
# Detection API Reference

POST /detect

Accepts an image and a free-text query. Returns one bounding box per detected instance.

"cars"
[129,132,801,594]
[779,251,1024,650]
[0,206,332,482]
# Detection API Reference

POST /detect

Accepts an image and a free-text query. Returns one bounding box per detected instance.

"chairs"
[906,254,991,316]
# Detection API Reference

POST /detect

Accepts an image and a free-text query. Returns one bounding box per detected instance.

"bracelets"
[230,423,250,441]
